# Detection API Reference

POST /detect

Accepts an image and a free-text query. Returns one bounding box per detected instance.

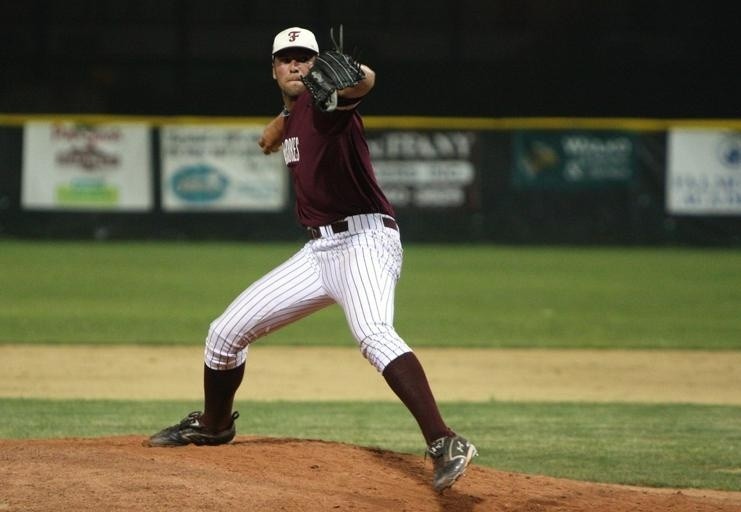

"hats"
[271,27,320,57]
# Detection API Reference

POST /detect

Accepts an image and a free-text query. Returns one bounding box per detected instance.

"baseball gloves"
[301,25,366,112]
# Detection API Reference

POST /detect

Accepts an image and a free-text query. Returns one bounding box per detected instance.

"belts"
[307,218,398,240]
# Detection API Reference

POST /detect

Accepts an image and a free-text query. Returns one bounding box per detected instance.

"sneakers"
[426,432,478,492]
[143,411,238,446]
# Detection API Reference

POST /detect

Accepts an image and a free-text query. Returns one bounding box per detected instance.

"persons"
[141,27,477,493]
